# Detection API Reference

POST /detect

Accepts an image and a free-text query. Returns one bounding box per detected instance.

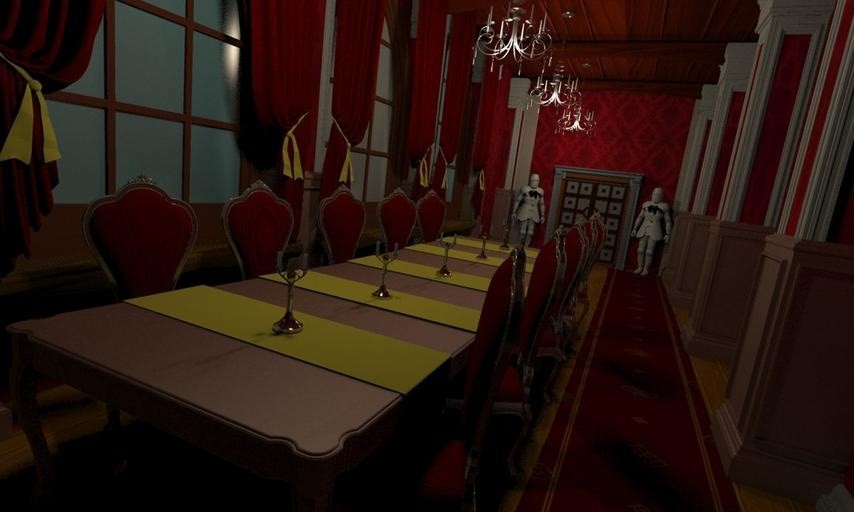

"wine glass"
[272,250,308,335]
[371,240,399,298]
[475,225,490,259]
[436,229,457,278]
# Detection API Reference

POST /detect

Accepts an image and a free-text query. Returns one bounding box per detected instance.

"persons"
[630,186,672,278]
[510,174,546,245]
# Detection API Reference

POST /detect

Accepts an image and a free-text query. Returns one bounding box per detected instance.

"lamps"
[469,0,599,136]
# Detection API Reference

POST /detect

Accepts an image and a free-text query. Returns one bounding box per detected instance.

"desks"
[3,232,550,510]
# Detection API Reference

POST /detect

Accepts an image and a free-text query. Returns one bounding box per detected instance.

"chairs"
[375,185,417,253]
[396,215,612,509]
[414,189,448,244]
[220,176,297,283]
[315,180,369,268]
[80,176,199,299]
